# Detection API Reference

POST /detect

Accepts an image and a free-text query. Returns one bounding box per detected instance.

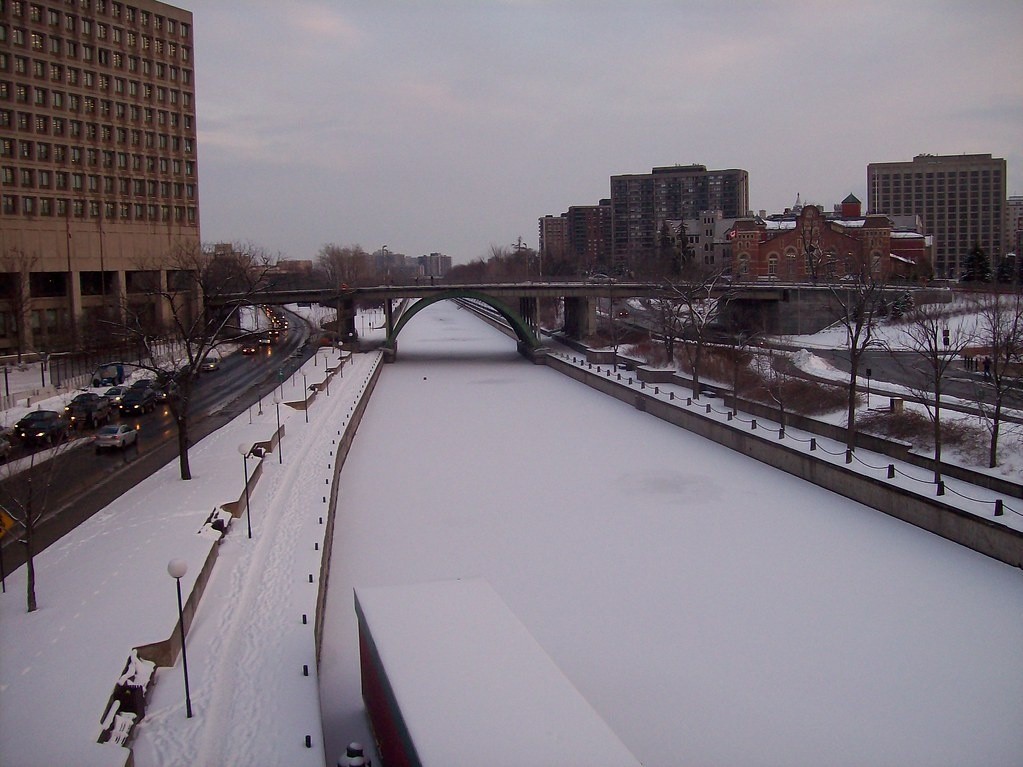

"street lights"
[382,245,387,282]
[349,333,353,365]
[99,227,107,301]
[273,396,281,464]
[339,342,342,378]
[238,444,252,539]
[324,353,329,396]
[523,243,529,281]
[361,316,364,337]
[66,223,75,318]
[678,240,682,262]
[167,558,192,718]
[302,370,308,424]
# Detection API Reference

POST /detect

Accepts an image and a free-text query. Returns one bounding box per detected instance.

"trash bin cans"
[889,396,903,414]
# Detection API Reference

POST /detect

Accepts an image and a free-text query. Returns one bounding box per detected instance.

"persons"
[982,356,992,377]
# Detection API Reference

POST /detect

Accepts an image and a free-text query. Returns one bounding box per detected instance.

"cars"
[619,309,628,316]
[258,334,271,346]
[14,393,112,444]
[131,379,177,400]
[707,275,731,284]
[118,390,156,416]
[201,358,220,372]
[0,437,12,458]
[588,274,617,284]
[94,425,138,452]
[262,304,289,337]
[960,274,992,284]
[180,365,200,379]
[102,386,130,407]
[243,344,257,355]
[840,274,864,284]
